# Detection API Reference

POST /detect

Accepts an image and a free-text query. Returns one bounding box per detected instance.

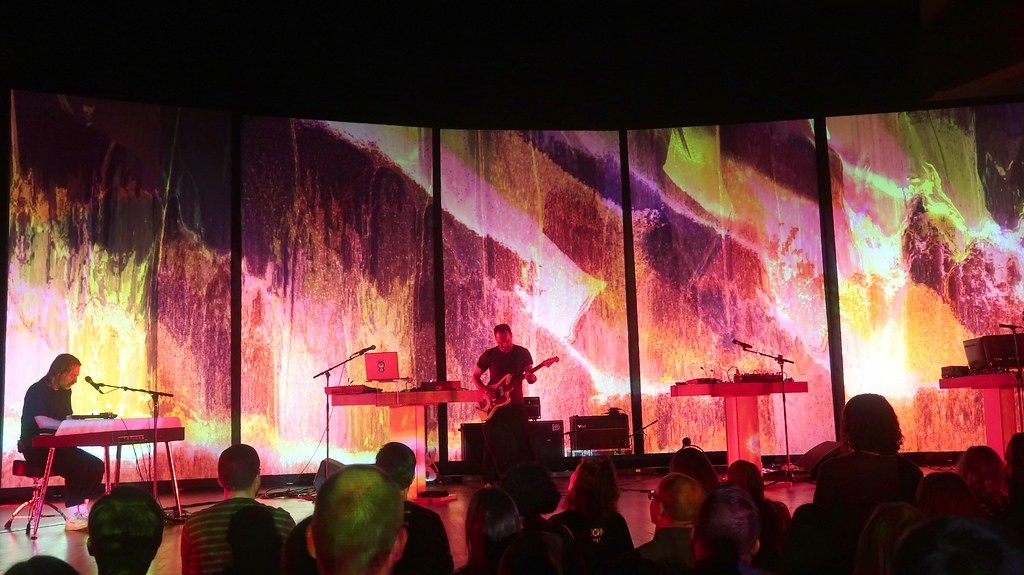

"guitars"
[477,355,560,421]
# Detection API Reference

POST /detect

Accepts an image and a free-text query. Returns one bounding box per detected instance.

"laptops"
[963,333,1024,370]
[365,352,410,380]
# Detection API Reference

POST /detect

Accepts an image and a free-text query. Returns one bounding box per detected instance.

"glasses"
[648,490,666,509]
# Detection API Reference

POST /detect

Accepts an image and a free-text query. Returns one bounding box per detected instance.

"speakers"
[462,420,564,464]
[525,396,540,419]
[569,414,629,450]
[313,458,346,489]
[796,441,845,477]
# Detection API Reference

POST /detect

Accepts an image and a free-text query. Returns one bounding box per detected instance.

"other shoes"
[64,513,89,531]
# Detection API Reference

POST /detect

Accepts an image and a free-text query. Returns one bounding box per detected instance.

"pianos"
[26,416,187,541]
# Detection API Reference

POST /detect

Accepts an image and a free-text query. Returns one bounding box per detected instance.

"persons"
[473,324,536,478]
[19,353,105,530]
[3,482,165,575]
[181,395,1024,575]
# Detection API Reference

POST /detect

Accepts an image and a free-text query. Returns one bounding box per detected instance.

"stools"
[5,461,69,535]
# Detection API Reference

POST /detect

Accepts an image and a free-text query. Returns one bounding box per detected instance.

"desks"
[332,390,480,499]
[940,373,1023,464]
[671,381,808,478]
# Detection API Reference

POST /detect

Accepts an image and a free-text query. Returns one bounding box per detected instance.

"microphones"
[85,375,103,394]
[999,324,1022,328]
[354,345,376,355]
[732,340,752,349]
